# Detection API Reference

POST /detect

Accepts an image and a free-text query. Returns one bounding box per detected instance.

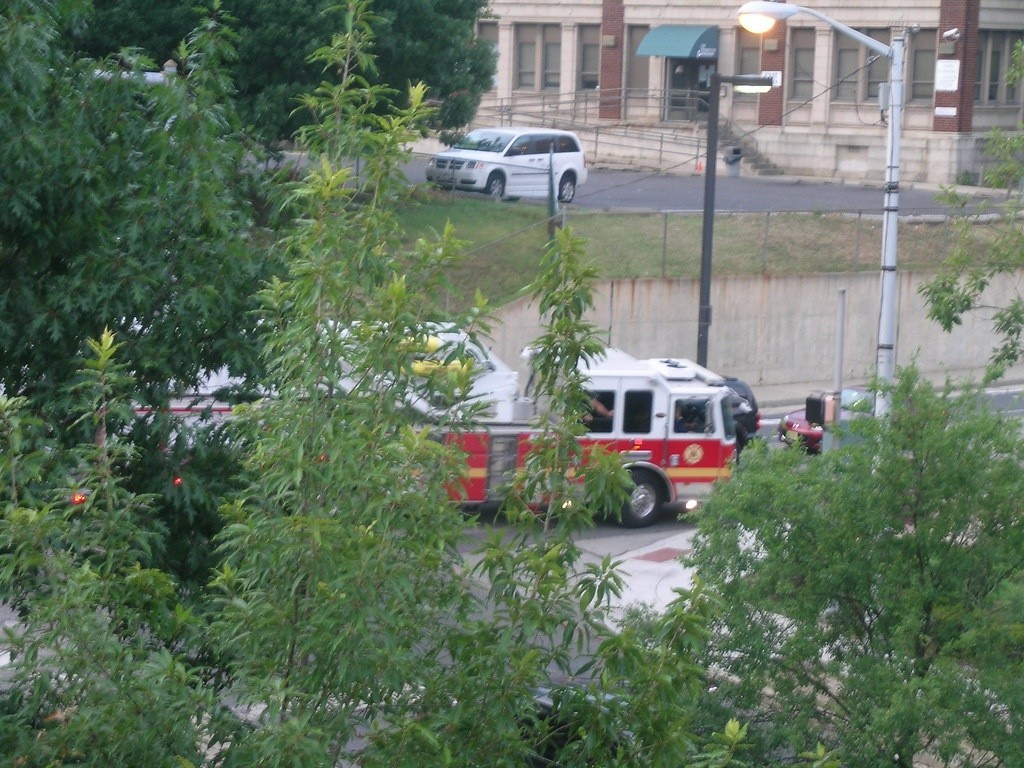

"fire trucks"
[99,317,756,532]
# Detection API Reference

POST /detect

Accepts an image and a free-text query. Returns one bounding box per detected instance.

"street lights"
[697,73,770,365]
[736,1,908,483]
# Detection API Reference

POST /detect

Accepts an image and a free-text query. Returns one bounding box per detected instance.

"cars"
[780,386,951,460]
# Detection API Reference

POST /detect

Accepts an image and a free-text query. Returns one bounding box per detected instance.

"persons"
[576,392,614,423]
[674,404,694,434]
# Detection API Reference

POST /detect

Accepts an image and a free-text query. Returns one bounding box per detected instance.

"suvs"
[427,127,587,203]
